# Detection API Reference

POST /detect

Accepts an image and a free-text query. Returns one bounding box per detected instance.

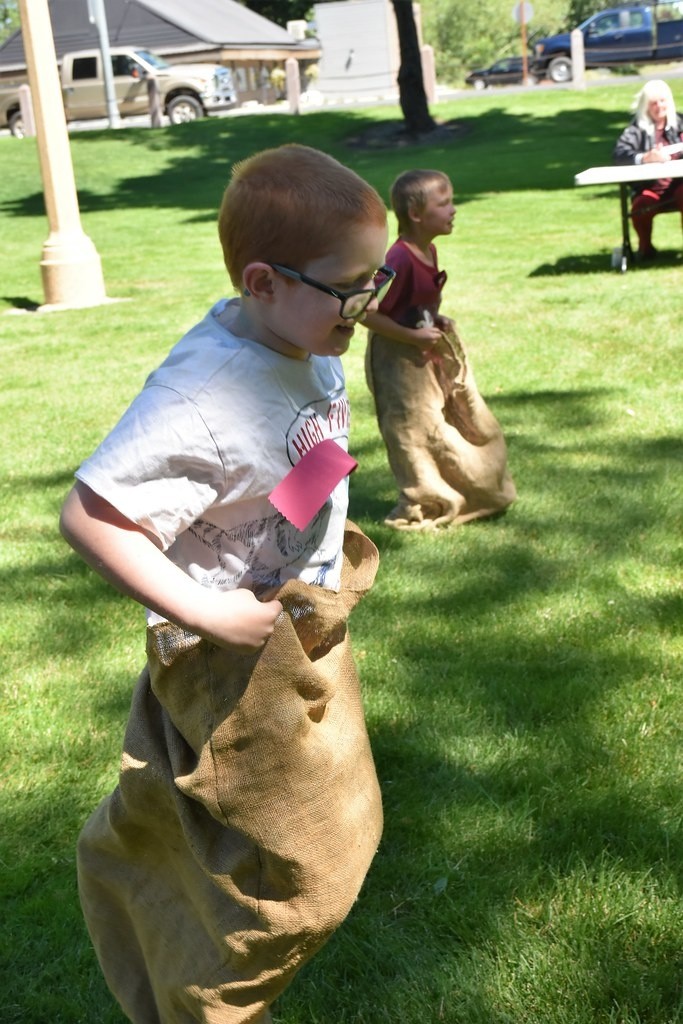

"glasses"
[241,265,396,319]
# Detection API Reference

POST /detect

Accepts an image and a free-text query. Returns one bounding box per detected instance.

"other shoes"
[639,244,657,259]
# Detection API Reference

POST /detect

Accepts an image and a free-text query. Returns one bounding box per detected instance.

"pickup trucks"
[0,48,236,137]
[534,0,683,84]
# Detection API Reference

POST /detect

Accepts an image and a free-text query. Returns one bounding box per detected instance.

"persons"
[614,80,683,264]
[365,169,457,394]
[59,143,395,655]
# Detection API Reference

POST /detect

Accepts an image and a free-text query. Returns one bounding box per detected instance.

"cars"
[465,55,538,90]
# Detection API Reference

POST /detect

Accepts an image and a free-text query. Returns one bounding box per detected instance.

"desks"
[573,160,683,272]
